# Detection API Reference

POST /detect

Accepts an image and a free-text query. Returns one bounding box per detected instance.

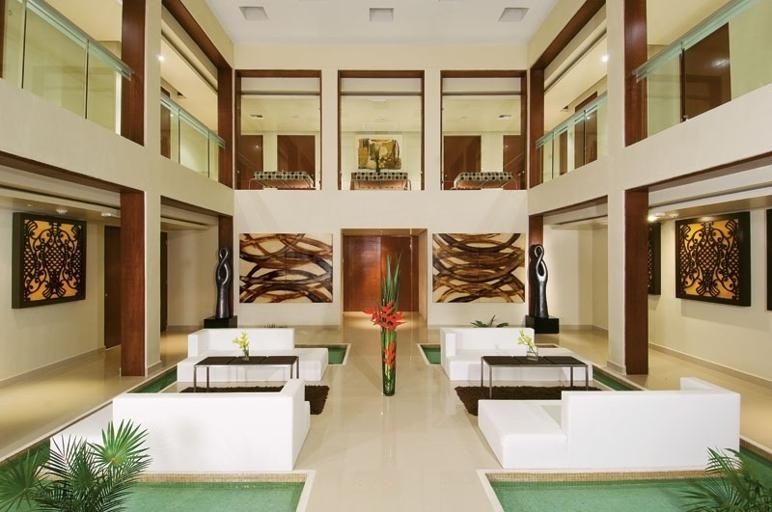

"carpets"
[454,385,603,414]
[179,385,331,414]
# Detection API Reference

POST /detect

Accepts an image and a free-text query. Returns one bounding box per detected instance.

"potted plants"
[231,330,251,360]
[362,248,406,396]
[517,328,539,360]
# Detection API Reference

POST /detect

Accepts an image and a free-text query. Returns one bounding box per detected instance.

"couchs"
[50,376,311,474]
[176,326,329,382]
[441,327,594,382]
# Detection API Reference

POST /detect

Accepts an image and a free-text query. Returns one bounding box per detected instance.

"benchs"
[477,375,741,470]
